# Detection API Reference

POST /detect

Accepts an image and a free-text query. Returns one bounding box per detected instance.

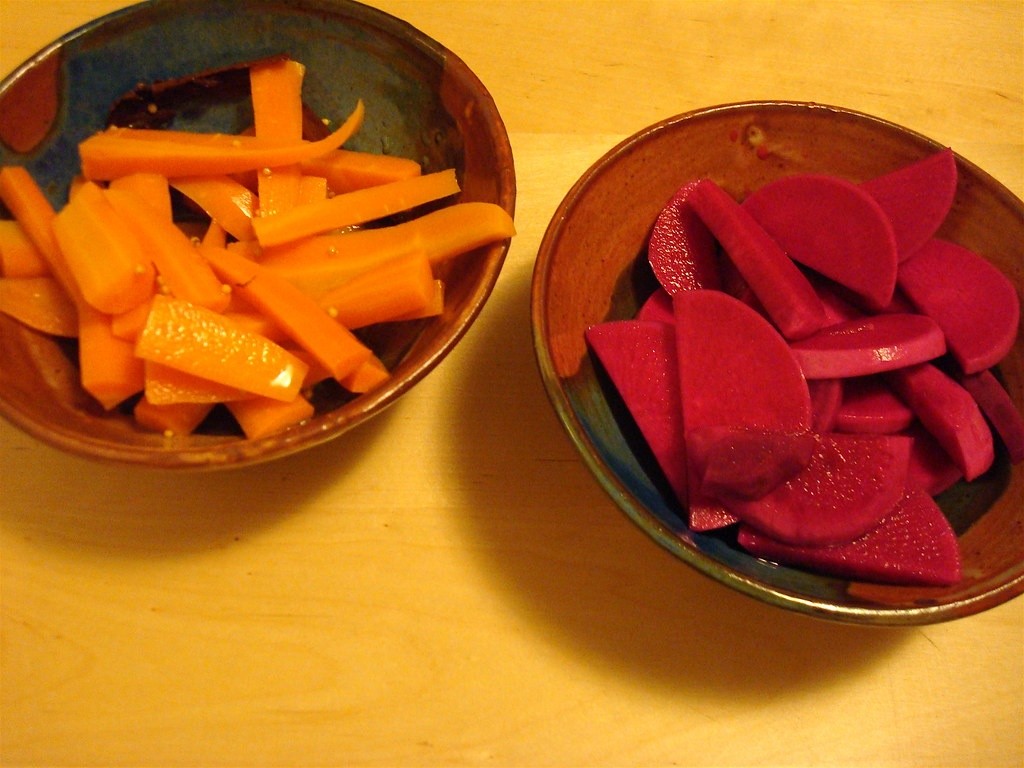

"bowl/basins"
[533,98,1024,626]
[0,1,514,472]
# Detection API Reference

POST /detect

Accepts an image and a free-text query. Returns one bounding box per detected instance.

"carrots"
[0,59,518,440]
